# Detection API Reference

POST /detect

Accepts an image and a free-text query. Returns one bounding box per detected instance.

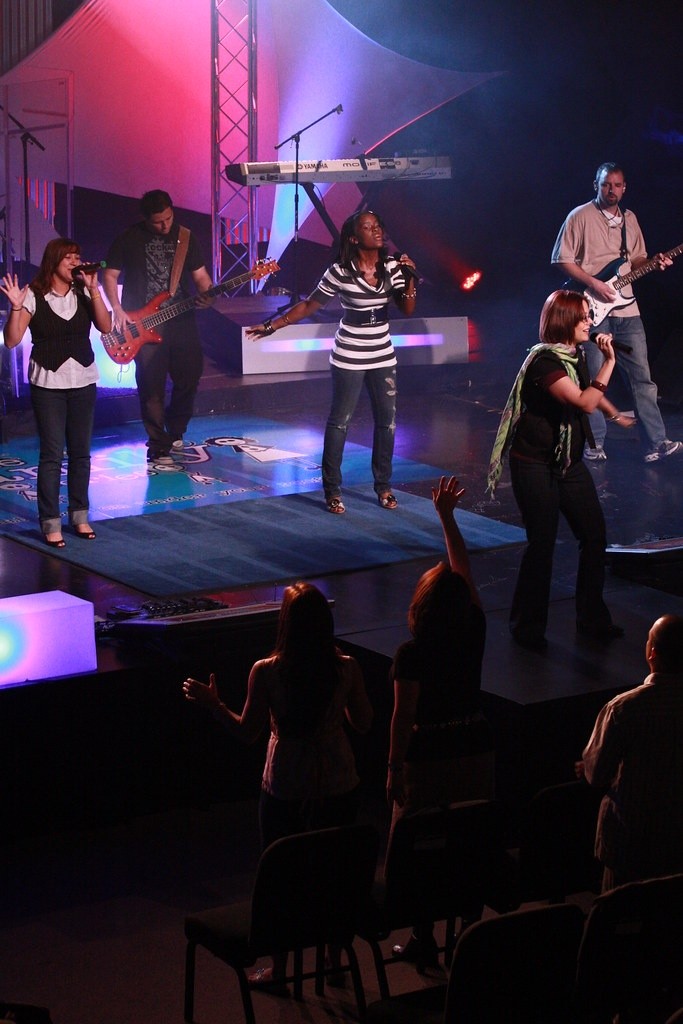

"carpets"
[3,478,528,597]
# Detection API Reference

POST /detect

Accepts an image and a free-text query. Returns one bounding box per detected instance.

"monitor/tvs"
[606,536,683,562]
[115,599,337,635]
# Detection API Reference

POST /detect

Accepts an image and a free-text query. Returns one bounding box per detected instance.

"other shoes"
[153,456,174,465]
[171,438,183,452]
[519,629,549,648]
[247,969,273,988]
[323,959,346,987]
[393,935,441,968]
[577,621,626,641]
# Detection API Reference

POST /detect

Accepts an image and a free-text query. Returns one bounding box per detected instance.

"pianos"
[224,155,452,293]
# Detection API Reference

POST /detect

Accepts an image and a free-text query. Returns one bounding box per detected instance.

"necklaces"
[597,199,618,219]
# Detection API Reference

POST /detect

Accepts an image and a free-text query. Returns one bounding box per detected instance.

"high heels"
[73,523,96,539]
[45,533,66,548]
[378,492,397,509]
[326,497,345,515]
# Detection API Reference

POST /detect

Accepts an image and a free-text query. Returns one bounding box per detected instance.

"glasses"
[579,313,593,325]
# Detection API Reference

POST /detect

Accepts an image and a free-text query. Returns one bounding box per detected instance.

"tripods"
[260,105,345,326]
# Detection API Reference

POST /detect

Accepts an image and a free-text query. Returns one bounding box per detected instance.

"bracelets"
[213,703,225,718]
[610,413,619,422]
[591,381,606,392]
[387,764,403,772]
[264,320,275,335]
[282,314,289,322]
[401,288,416,299]
[91,293,100,299]
[11,307,22,311]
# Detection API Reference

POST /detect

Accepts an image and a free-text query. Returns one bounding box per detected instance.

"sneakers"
[584,444,607,464]
[644,439,682,463]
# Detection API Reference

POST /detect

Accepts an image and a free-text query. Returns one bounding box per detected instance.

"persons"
[102,190,217,465]
[550,161,683,464]
[0,238,112,550]
[575,614,683,893]
[383,475,486,963]
[183,582,374,989]
[246,212,417,514]
[509,290,638,647]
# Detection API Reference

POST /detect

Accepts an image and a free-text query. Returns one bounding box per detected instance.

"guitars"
[560,244,683,333]
[100,256,282,364]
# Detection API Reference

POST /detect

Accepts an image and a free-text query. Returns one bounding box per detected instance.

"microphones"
[590,332,634,354]
[73,261,107,277]
[393,251,424,285]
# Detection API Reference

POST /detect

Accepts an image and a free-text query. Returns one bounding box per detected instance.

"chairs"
[182,780,683,1024]
